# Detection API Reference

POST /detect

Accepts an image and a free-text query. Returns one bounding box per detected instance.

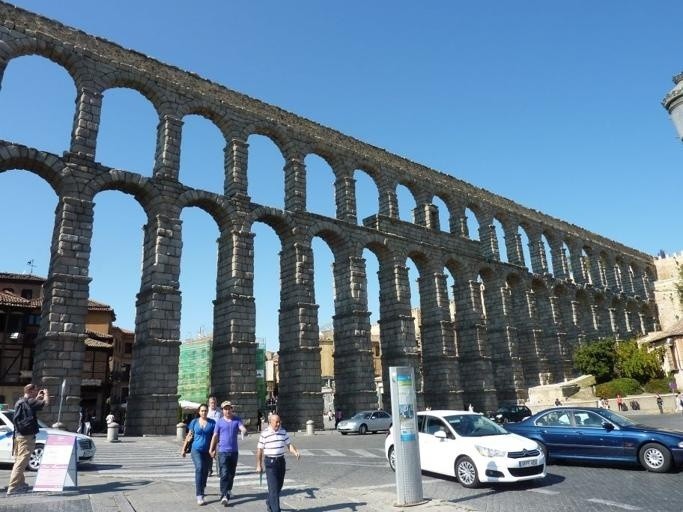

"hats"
[221,401,231,408]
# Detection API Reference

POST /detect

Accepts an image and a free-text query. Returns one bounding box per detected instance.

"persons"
[468,403,474,412]
[555,398,562,406]
[257,409,263,431]
[425,405,432,411]
[7,383,50,495]
[328,410,333,421]
[180,396,247,507]
[656,392,683,414]
[76,407,94,437]
[335,408,342,430]
[105,411,115,426]
[257,413,300,512]
[599,394,622,412]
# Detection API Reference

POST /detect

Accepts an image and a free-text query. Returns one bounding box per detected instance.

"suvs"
[485,404,533,424]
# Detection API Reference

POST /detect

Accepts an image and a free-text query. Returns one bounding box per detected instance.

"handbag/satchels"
[184,431,194,453]
[14,401,38,434]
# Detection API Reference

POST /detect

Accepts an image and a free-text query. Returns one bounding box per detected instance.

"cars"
[256,400,276,424]
[502,405,682,475]
[337,409,393,436]
[383,410,550,488]
[0,402,96,472]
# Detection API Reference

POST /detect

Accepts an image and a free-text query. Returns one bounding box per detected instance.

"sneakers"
[221,496,229,506]
[7,483,33,494]
[197,495,204,505]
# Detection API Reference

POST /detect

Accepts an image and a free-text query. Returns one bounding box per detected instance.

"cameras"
[40,391,44,394]
